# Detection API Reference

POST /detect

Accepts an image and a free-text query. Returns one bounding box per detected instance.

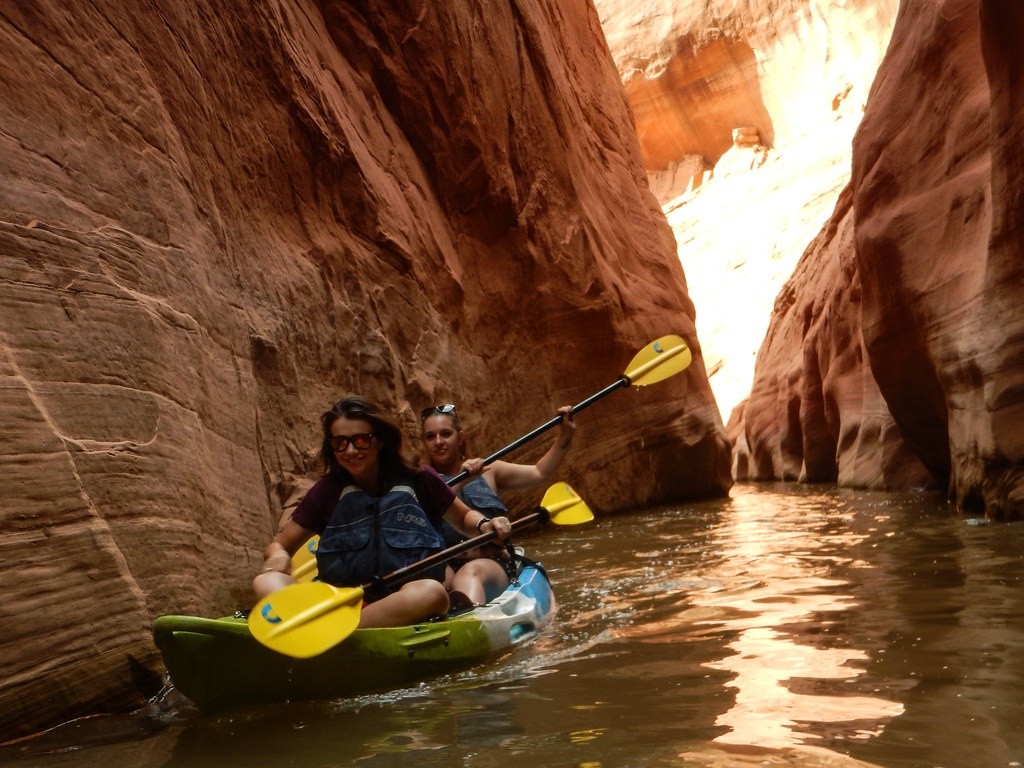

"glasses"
[327,432,378,452]
[420,405,459,427]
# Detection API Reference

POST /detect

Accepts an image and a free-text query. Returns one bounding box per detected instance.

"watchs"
[474,516,491,533]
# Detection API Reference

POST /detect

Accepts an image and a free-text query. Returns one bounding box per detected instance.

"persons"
[412,402,579,611]
[255,396,510,629]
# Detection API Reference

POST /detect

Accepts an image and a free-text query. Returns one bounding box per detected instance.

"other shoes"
[448,590,474,616]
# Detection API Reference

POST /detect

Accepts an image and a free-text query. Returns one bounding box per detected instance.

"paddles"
[248,478,599,663]
[279,333,694,588]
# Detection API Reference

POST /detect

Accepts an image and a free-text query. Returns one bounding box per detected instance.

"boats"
[151,545,557,716]
